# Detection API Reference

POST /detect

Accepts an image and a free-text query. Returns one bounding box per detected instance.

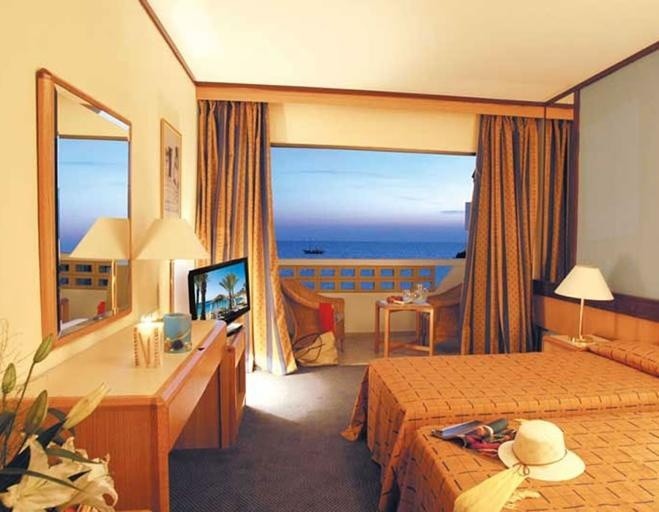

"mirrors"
[35,66,133,352]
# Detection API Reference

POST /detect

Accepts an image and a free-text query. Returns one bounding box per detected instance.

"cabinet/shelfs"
[228,324,253,447]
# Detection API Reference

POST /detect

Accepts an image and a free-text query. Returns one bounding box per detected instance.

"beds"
[337,338,659,512]
[377,409,659,512]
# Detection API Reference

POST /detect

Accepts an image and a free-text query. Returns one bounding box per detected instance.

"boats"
[302,246,326,255]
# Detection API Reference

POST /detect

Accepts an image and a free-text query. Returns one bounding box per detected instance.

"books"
[431,418,517,463]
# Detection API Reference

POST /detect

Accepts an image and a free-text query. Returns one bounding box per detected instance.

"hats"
[499,418,586,481]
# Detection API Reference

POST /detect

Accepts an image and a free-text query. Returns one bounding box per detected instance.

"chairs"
[421,281,462,355]
[279,276,346,353]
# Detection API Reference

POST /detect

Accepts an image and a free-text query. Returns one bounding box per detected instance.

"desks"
[0,317,228,511]
[373,298,435,358]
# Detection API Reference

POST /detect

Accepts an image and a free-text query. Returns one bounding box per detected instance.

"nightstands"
[541,331,612,352]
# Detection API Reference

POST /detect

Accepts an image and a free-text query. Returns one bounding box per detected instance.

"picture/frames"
[159,117,183,222]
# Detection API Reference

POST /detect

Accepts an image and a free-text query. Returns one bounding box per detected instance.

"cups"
[164,312,193,354]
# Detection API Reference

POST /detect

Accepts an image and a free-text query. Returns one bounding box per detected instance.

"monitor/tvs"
[188,257,250,335]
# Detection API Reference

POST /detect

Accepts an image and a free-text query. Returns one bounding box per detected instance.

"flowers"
[0,316,121,511]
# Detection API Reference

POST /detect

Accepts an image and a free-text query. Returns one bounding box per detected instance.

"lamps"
[133,217,211,313]
[66,216,130,316]
[552,263,616,343]
